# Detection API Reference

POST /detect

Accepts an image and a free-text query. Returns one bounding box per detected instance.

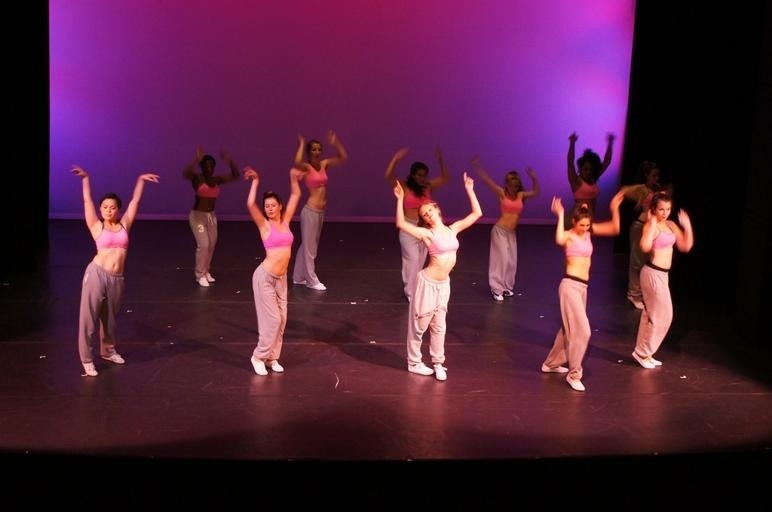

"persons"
[632,186,695,368]
[183,147,240,286]
[394,171,483,380]
[469,153,539,303]
[565,131,613,230]
[242,167,304,376]
[295,131,348,292]
[69,166,161,377]
[621,165,672,310]
[385,144,449,304]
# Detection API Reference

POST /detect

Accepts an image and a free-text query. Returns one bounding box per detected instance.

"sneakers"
[197,273,215,287]
[433,364,447,380]
[82,362,98,376]
[307,283,327,290]
[293,280,308,285]
[632,351,663,369]
[492,291,503,300]
[102,354,125,364]
[627,296,644,310]
[566,374,585,391]
[408,362,434,375]
[266,360,284,372]
[542,364,569,373]
[504,289,514,297]
[251,356,268,375]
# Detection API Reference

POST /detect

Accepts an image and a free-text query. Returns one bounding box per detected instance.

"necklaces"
[541,191,627,391]
